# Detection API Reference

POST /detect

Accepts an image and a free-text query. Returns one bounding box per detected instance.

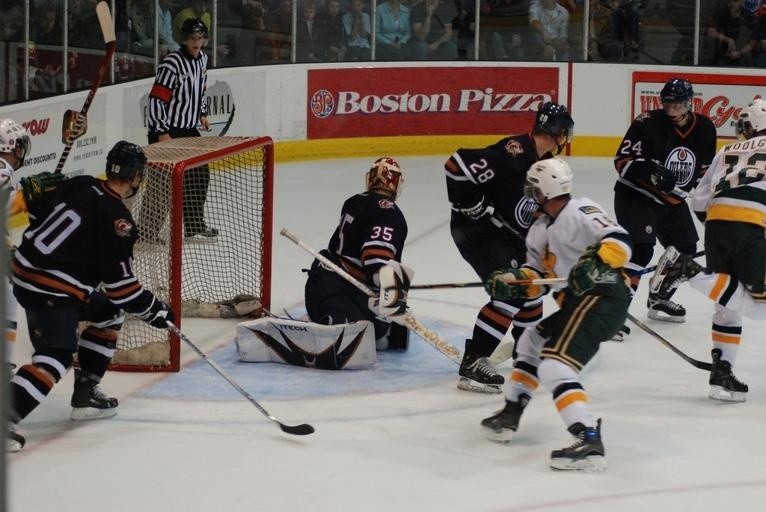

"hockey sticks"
[166,321,314,435]
[55,2,117,174]
[626,311,713,371]
[280,227,516,368]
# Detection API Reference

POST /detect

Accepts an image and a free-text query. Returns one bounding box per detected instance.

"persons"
[0,1,765,110]
[615,78,719,334]
[1,118,31,377]
[444,103,576,383]
[0,141,171,451]
[305,158,410,350]
[482,159,633,459]
[692,100,765,392]
[134,19,218,244]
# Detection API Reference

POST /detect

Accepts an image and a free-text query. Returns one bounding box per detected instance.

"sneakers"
[135,227,166,247]
[185,225,218,238]
[4,419,25,451]
[71,383,118,408]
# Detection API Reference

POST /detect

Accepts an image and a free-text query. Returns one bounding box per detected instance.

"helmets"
[105,141,148,182]
[182,16,206,41]
[0,118,31,154]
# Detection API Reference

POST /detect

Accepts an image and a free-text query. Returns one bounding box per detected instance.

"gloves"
[135,296,176,329]
